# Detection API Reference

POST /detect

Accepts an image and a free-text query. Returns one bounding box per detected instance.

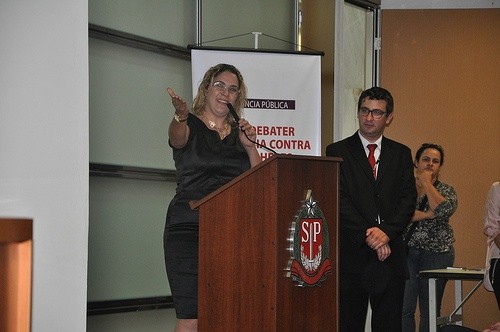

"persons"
[163,65,261,332]
[325,87,418,332]
[401,144,457,332]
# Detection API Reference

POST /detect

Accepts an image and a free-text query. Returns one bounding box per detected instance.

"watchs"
[173,111,190,125]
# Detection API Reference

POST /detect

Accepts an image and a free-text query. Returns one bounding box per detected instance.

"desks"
[418,268,485,332]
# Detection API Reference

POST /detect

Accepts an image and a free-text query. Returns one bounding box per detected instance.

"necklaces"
[200,110,229,141]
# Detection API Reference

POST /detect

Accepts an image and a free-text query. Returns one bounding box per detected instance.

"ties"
[366,144,378,177]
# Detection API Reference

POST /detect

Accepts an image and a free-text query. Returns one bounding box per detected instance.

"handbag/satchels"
[400,240,409,280]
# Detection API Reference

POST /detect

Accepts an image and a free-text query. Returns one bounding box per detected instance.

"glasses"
[358,108,390,119]
[209,81,239,96]
[422,143,441,150]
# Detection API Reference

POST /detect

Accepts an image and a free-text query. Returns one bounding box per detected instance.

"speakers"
[344,0,381,11]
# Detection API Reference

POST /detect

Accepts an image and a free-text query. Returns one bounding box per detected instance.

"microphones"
[227,103,276,154]
